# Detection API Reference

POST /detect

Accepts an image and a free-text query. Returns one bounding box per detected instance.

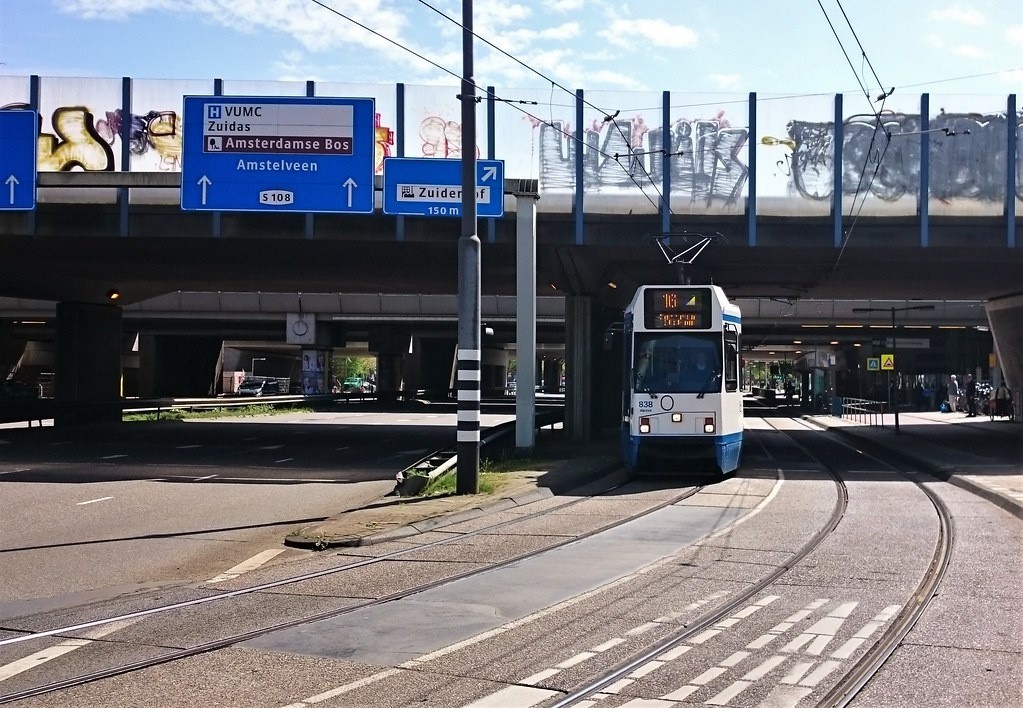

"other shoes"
[966,414,972,417]
[972,415,976,417]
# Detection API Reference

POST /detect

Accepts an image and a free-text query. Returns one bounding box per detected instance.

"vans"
[344,377,361,389]
[244,376,279,394]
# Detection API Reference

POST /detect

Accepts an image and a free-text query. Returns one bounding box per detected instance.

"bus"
[601,232,746,481]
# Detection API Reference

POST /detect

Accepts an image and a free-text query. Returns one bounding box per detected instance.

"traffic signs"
[180,94,375,214]
[383,154,503,218]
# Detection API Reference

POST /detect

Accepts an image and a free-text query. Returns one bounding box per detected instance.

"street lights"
[251,357,266,376]
[851,304,935,432]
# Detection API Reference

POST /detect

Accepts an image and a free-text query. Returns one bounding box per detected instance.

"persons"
[690,350,714,380]
[947,375,959,412]
[361,386,365,393]
[915,383,923,411]
[784,382,795,406]
[748,379,782,391]
[370,386,374,392]
[966,374,976,417]
[923,386,930,410]
[996,382,1013,413]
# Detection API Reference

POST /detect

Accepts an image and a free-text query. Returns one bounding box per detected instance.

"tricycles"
[987,386,1015,422]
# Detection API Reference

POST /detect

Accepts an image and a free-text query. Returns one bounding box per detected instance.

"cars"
[235,378,268,396]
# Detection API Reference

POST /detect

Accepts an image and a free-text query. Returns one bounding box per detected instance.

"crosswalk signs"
[868,359,879,369]
